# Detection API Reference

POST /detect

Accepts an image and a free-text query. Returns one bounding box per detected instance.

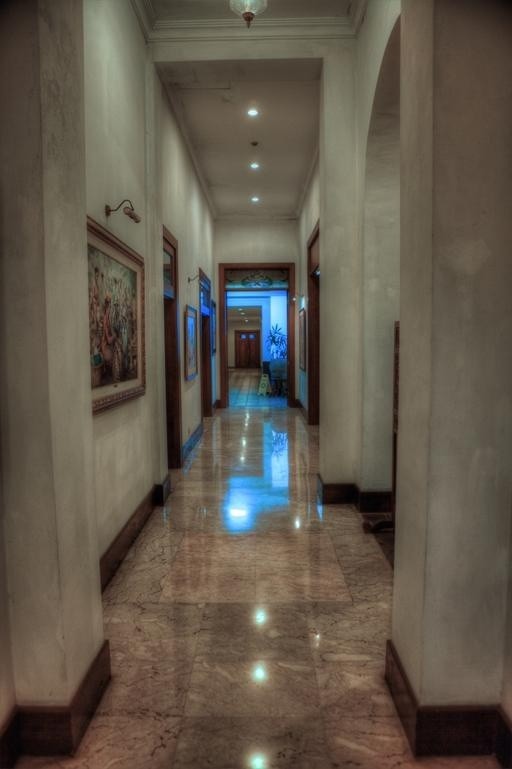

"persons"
[100,294,117,363]
[91,266,99,323]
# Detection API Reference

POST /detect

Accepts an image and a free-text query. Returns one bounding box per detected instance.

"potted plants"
[265,323,289,380]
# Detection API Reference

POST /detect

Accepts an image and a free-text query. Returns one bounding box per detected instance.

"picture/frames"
[185,304,198,380]
[298,308,307,371]
[86,213,145,415]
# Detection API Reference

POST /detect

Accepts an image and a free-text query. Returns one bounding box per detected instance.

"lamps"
[104,200,141,223]
